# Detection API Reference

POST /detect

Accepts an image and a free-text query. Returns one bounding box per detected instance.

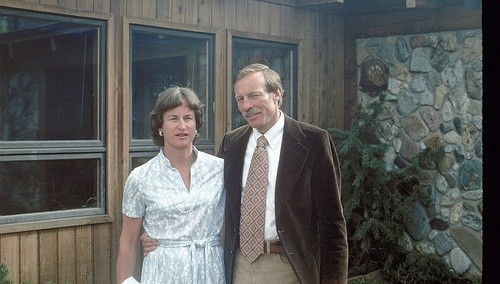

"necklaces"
[162,158,201,212]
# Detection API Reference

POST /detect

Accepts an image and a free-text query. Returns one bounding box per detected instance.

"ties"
[240,135,269,263]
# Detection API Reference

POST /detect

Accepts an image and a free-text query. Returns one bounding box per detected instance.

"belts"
[264,242,286,254]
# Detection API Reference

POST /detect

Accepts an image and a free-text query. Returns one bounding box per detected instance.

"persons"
[141,56,347,284]
[116,83,227,284]
